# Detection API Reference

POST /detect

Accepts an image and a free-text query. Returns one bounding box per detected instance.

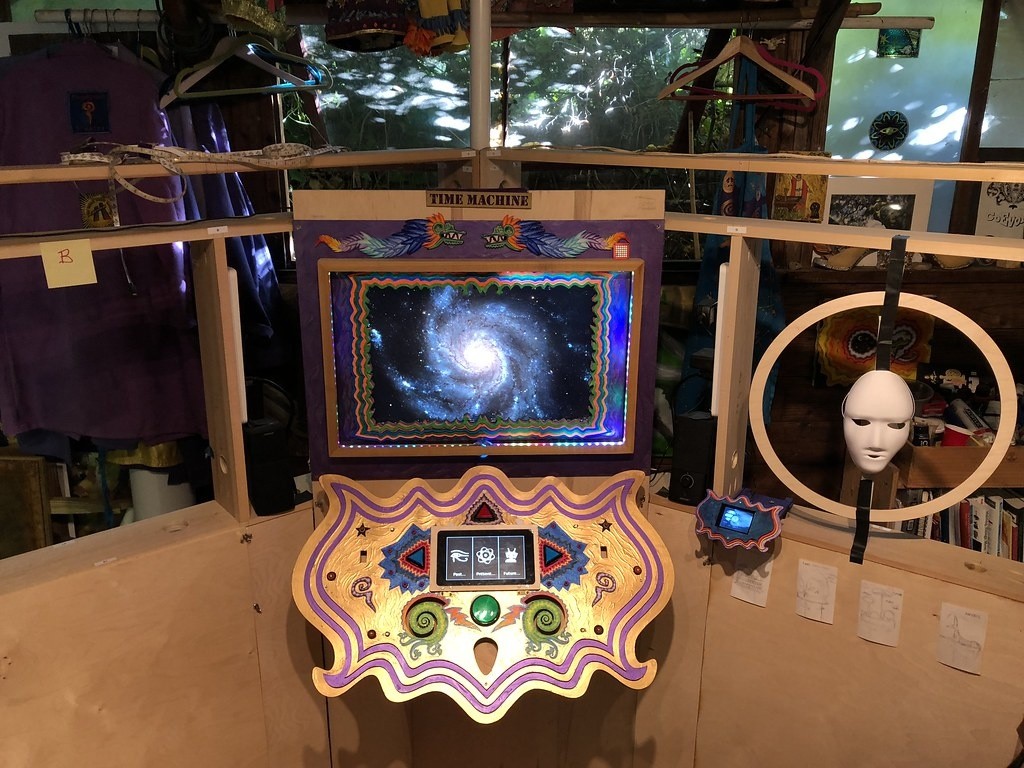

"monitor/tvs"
[318,256,645,458]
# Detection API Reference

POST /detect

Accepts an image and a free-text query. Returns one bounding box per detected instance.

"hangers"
[56,12,334,111]
[657,15,827,113]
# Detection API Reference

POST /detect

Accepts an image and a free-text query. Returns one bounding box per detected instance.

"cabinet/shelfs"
[839,434,1024,564]
